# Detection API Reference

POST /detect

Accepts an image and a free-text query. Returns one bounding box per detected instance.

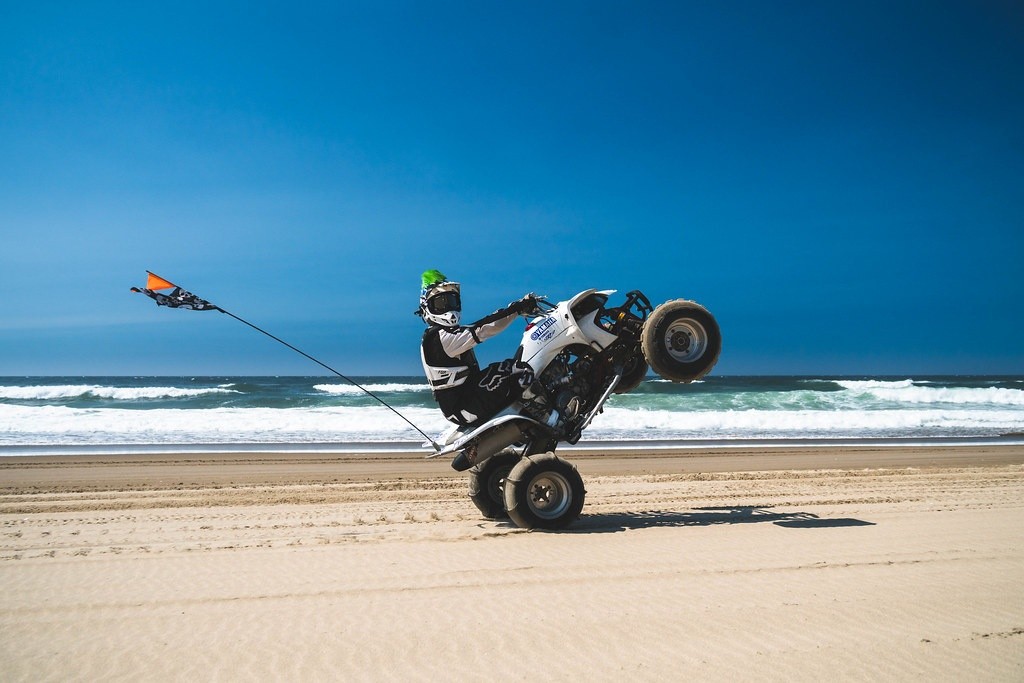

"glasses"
[431,293,460,310]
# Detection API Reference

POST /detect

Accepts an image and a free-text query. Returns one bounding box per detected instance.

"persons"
[415,269,581,433]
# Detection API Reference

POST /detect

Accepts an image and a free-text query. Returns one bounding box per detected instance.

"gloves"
[520,293,540,318]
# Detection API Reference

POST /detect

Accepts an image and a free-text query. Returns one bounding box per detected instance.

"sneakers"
[550,396,581,438]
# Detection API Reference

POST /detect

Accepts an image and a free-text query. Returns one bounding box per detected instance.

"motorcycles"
[421,289,724,531]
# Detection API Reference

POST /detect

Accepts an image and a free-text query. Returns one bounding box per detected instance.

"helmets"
[414,270,462,331]
